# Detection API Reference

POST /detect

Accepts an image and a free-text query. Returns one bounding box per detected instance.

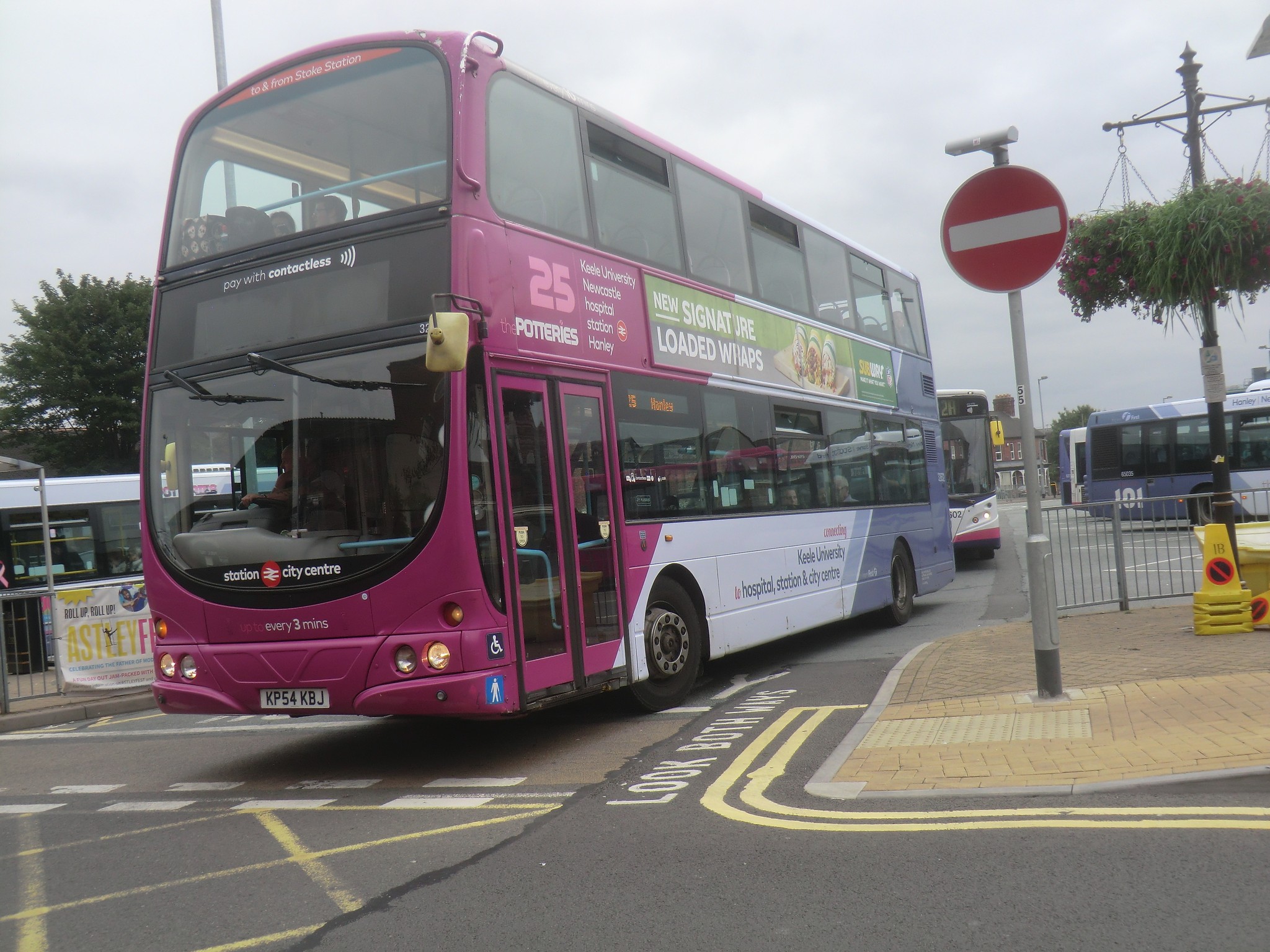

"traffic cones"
[1189,522,1253,636]
[1248,589,1270,627]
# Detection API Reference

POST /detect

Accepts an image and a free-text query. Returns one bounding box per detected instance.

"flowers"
[1056,178,1270,325]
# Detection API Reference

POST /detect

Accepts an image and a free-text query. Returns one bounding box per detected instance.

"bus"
[511,428,927,586]
[936,387,1004,561]
[1057,429,1072,505]
[1083,379,1270,526]
[133,32,958,722]
[1068,426,1088,512]
[0,462,284,674]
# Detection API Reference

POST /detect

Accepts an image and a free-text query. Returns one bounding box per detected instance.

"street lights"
[1162,396,1173,403]
[894,287,920,356]
[0,456,55,592]
[1037,376,1052,496]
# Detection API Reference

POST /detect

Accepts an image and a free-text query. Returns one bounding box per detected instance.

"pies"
[792,324,837,388]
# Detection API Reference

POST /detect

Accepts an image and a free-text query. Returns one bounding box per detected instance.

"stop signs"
[939,164,1069,294]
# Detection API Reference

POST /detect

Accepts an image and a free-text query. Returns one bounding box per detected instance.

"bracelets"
[274,486,277,489]
[261,494,267,498]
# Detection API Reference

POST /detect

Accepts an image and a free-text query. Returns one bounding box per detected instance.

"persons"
[241,443,344,532]
[126,546,143,572]
[51,542,85,573]
[106,548,126,574]
[834,475,859,502]
[779,483,798,505]
[120,586,147,612]
[312,196,347,228]
[270,211,296,236]
